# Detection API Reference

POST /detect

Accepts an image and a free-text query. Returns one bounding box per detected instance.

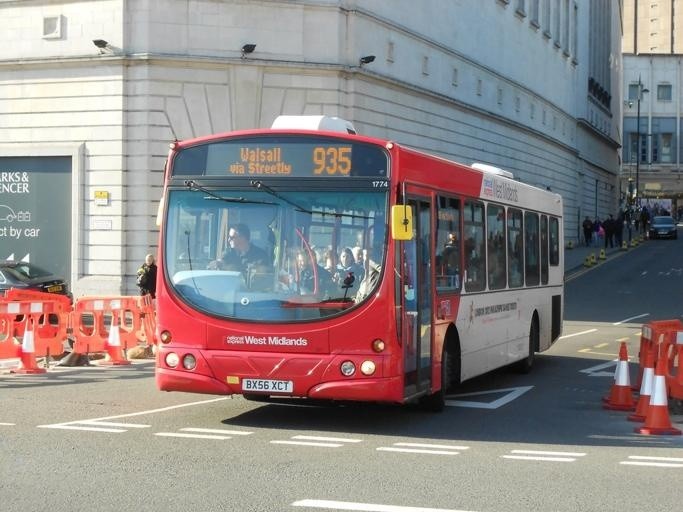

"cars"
[0,259,73,308]
[647,216,679,240]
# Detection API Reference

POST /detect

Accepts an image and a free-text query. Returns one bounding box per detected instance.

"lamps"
[91,39,108,49]
[360,56,376,64]
[241,43,256,54]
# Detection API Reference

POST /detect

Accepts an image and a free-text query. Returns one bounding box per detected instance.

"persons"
[613,217,624,248]
[600,213,615,248]
[135,254,157,299]
[205,222,269,286]
[592,215,603,249]
[282,226,552,296]
[582,215,592,247]
[617,202,682,231]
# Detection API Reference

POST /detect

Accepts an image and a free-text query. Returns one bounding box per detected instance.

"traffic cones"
[603,340,683,436]
[96,309,130,365]
[10,316,47,375]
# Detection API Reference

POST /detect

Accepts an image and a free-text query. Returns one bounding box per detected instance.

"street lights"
[636,88,649,195]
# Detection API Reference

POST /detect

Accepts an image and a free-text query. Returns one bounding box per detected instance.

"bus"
[154,116,565,412]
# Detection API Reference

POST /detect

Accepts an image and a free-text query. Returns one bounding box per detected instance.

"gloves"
[138,268,145,273]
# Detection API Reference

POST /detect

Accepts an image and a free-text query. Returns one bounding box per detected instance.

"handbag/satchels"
[136,271,147,286]
[598,226,605,237]
